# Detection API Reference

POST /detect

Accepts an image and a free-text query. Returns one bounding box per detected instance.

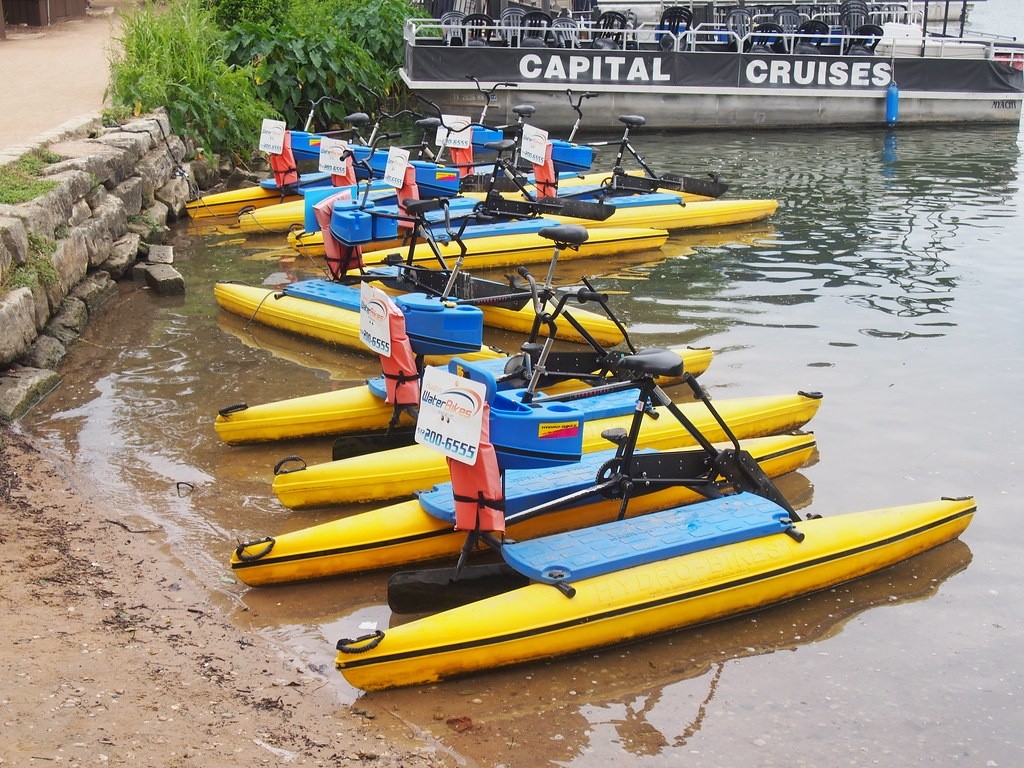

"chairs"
[590,11,637,50]
[726,0,906,55]
[441,7,582,49]
[658,7,692,52]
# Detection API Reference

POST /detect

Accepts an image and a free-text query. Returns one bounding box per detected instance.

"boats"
[184,75,978,690]
[398,0,1023,133]
